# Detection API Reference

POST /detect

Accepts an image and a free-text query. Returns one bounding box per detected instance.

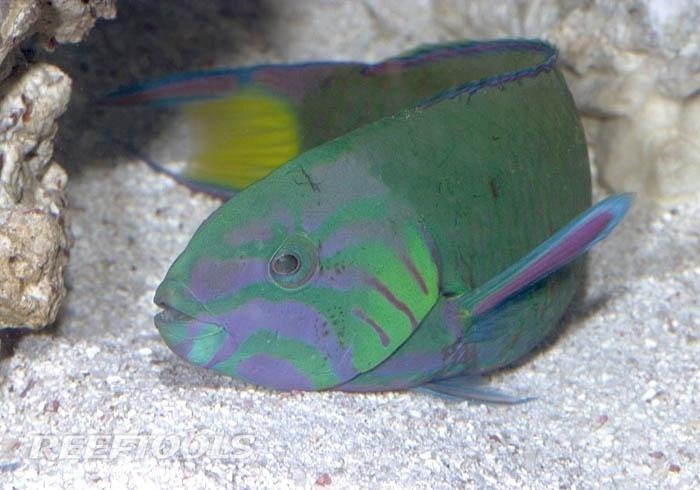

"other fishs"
[88,37,637,408]
